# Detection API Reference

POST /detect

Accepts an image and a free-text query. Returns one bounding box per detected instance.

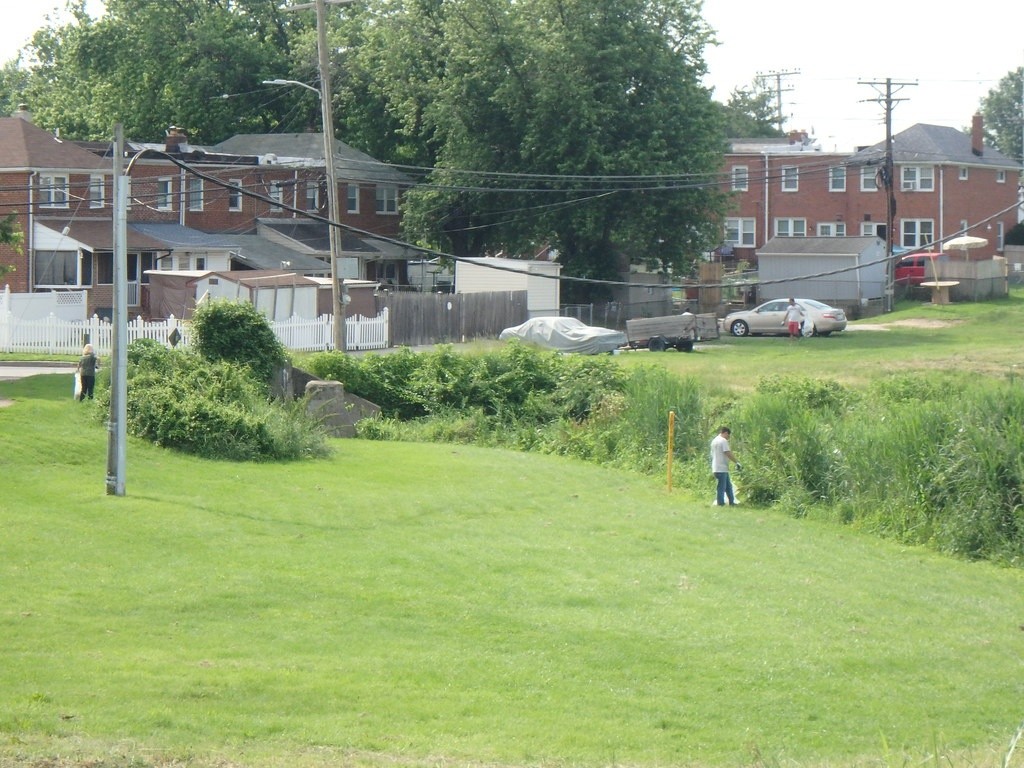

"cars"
[497,316,629,356]
[723,298,848,337]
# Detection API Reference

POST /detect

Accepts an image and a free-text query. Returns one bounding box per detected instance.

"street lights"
[262,78,347,351]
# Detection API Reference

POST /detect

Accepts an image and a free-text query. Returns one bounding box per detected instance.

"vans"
[895,252,949,287]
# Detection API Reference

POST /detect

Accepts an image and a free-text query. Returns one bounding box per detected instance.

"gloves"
[736,462,742,471]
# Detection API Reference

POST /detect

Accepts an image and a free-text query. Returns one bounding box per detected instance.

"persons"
[682,307,692,315]
[76,344,100,403]
[711,427,742,506]
[781,297,805,340]
